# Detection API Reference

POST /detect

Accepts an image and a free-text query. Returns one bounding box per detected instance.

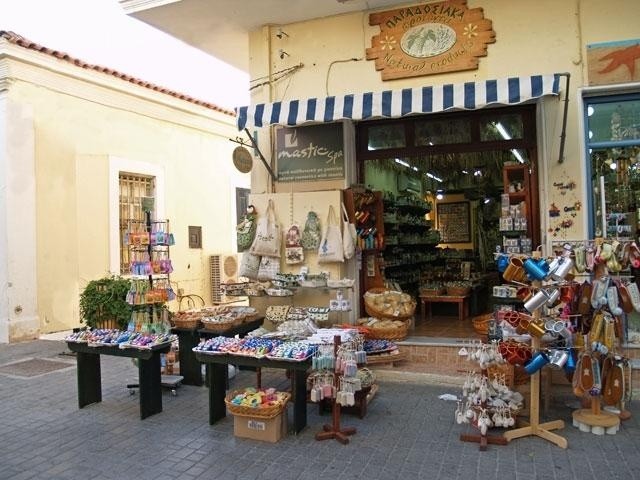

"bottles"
[354,208,385,249]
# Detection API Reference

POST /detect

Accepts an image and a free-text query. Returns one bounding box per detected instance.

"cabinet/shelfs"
[364,190,432,291]
[502,161,540,252]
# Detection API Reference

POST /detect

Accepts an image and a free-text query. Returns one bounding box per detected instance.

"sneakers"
[573,240,640,406]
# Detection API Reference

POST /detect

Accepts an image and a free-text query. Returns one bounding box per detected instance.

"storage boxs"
[232,407,288,444]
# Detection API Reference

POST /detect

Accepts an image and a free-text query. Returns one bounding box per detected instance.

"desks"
[193,353,315,436]
[169,315,265,388]
[66,339,171,420]
[418,295,469,322]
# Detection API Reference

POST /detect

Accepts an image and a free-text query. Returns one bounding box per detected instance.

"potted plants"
[77,275,131,331]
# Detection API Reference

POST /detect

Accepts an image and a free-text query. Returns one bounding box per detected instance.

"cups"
[492,250,580,375]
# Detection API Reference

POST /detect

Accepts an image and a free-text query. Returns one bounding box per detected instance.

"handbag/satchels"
[235,199,358,288]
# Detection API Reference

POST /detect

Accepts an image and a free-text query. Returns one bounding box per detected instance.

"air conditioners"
[207,252,242,305]
[397,173,423,194]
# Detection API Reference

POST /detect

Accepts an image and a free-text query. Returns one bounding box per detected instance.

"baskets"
[273,305,322,330]
[419,285,447,295]
[472,313,494,334]
[224,389,292,419]
[364,287,417,321]
[446,287,470,296]
[170,294,259,332]
[360,320,411,342]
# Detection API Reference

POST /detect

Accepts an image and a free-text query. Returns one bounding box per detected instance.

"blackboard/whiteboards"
[277,121,346,183]
